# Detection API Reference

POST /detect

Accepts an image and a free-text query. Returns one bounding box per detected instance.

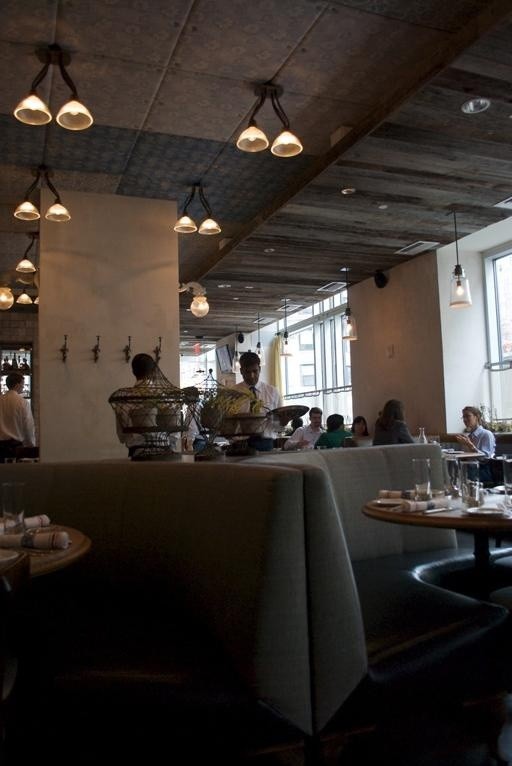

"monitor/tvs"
[215,344,232,370]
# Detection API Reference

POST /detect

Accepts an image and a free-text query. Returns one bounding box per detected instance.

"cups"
[0,504,33,540]
[374,426,512,519]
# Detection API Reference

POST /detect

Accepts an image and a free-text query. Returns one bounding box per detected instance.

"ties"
[249,387,256,396]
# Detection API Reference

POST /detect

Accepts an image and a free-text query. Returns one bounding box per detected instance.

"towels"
[0,514,69,550]
[379,489,451,512]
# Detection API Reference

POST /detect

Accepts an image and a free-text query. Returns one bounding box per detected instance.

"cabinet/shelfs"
[0,342,33,399]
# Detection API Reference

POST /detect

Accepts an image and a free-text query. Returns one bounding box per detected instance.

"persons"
[282,406,328,450]
[314,414,353,450]
[373,399,416,446]
[227,353,296,452]
[350,416,373,448]
[184,387,208,452]
[115,352,180,461]
[283,415,304,435]
[0,373,36,462]
[455,405,497,483]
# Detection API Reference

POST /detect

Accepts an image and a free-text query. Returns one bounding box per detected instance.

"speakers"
[374,272,388,288]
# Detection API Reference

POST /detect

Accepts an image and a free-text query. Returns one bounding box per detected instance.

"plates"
[267,405,310,421]
[0,548,21,566]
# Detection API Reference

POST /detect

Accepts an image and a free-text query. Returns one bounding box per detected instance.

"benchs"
[344,434,512,458]
[0,463,312,766]
[241,443,512,732]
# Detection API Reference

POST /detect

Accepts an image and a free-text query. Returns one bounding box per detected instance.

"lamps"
[173,182,221,235]
[190,282,210,317]
[279,300,293,356]
[449,215,472,308]
[0,234,40,310]
[254,312,265,367]
[341,266,357,340]
[14,52,94,131]
[13,170,71,222]
[232,326,241,374]
[236,84,303,158]
